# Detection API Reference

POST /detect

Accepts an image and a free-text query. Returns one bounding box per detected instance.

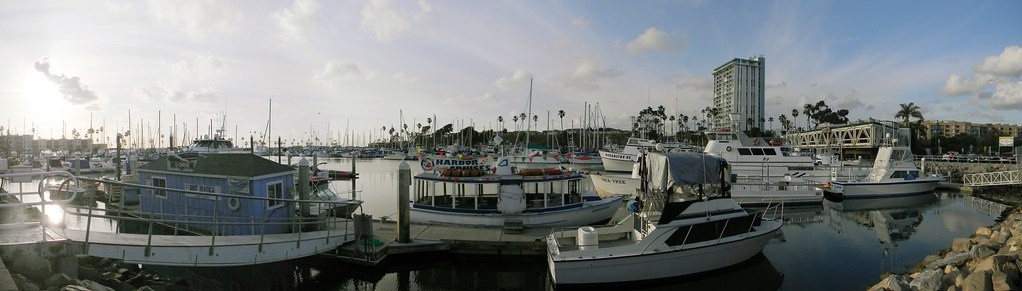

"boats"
[0,93,676,228]
[825,138,942,198]
[692,129,825,209]
[544,152,785,289]
[64,99,371,268]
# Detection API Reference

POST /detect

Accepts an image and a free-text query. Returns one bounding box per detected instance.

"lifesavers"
[753,138,760,146]
[227,197,240,211]
